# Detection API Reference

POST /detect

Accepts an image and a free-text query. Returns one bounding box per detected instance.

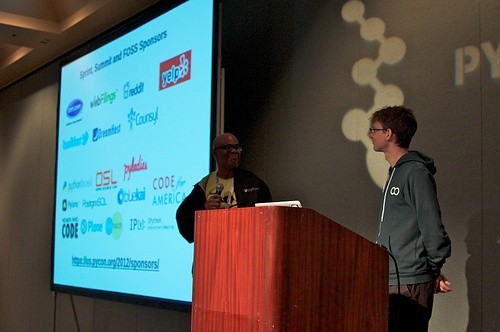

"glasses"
[369,128,398,135]
[214,145,242,151]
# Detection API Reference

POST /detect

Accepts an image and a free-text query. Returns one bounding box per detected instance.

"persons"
[369,106,453,332]
[176,132,274,277]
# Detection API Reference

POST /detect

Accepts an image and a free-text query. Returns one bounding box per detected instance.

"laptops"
[255,200,303,208]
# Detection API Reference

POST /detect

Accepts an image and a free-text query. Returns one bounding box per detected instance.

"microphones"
[216,183,224,195]
